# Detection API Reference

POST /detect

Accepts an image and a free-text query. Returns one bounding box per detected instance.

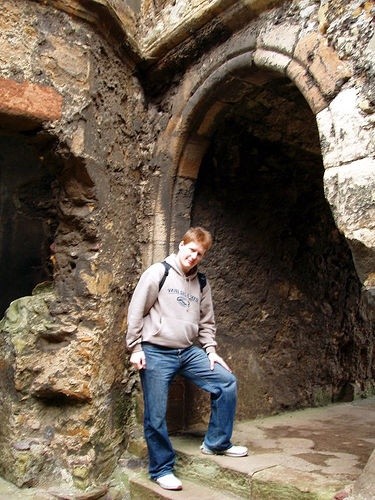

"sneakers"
[200,441,249,457]
[157,472,182,491]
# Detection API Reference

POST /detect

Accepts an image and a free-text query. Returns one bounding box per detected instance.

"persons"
[126,227,248,489]
